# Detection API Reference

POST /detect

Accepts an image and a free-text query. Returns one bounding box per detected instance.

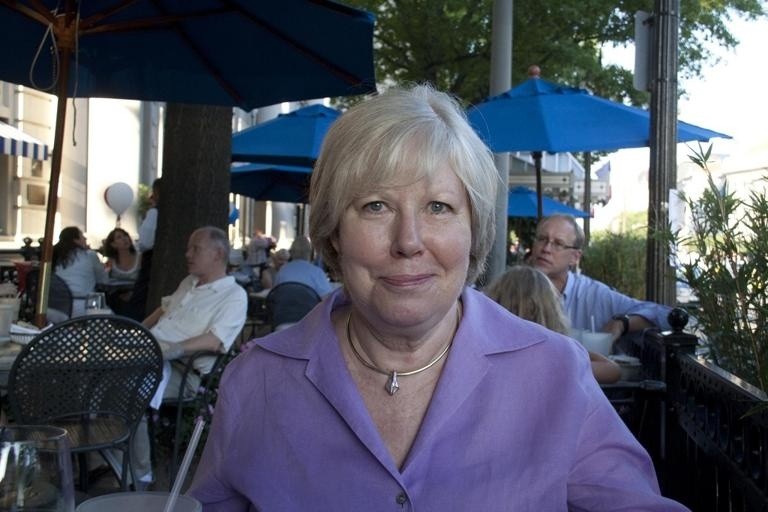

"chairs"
[596,379,667,450]
[0,242,348,511]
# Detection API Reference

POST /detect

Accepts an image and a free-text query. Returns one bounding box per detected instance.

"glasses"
[530,235,580,251]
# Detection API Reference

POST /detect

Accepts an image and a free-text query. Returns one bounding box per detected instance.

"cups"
[581,332,611,358]
[86,292,107,311]
[0,424,73,511]
[74,490,203,512]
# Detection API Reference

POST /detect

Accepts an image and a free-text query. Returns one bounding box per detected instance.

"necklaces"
[345,311,462,395]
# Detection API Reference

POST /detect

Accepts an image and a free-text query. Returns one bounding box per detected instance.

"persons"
[533,214,676,356]
[488,265,621,383]
[185,87,688,512]
[274,237,332,302]
[53,226,110,324]
[229,231,291,290]
[103,228,143,282]
[112,226,248,495]
[130,180,164,323]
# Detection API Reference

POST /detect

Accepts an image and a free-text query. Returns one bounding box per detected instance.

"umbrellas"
[508,185,592,219]
[0,0,379,328]
[464,62,735,219]
[228,104,344,237]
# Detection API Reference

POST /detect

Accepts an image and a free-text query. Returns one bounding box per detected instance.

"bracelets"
[612,313,629,335]
